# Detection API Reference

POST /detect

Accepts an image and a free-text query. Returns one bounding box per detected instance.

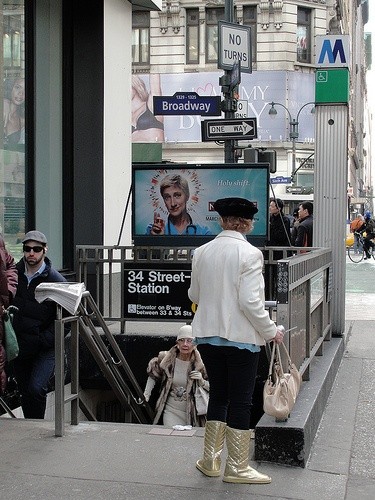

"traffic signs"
[203,117,257,141]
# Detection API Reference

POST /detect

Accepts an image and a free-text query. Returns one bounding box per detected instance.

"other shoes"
[364,257,371,260]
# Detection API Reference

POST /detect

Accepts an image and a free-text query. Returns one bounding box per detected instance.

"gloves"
[189,371,206,386]
[3,306,19,321]
[139,390,151,406]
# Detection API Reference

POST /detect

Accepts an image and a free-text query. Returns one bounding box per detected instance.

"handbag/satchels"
[194,381,209,415]
[263,339,303,418]
[3,309,19,362]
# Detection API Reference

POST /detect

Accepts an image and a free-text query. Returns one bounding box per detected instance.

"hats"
[22,231,48,244]
[214,197,259,220]
[177,325,196,339]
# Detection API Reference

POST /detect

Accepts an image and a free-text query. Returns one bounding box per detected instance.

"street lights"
[268,101,316,194]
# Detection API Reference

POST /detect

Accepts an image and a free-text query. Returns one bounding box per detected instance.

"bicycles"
[348,227,375,263]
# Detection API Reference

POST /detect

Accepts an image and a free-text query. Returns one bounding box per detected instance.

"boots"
[196,420,227,476]
[223,426,272,483]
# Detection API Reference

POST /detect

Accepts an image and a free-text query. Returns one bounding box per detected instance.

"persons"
[130,72,165,143]
[269,198,291,259]
[8,230,67,420]
[146,173,212,235]
[1,78,25,146]
[139,324,211,428]
[290,202,313,257]
[0,232,18,395]
[186,198,285,484]
[349,212,375,260]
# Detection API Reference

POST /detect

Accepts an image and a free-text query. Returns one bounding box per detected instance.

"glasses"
[178,339,193,344]
[23,246,44,252]
